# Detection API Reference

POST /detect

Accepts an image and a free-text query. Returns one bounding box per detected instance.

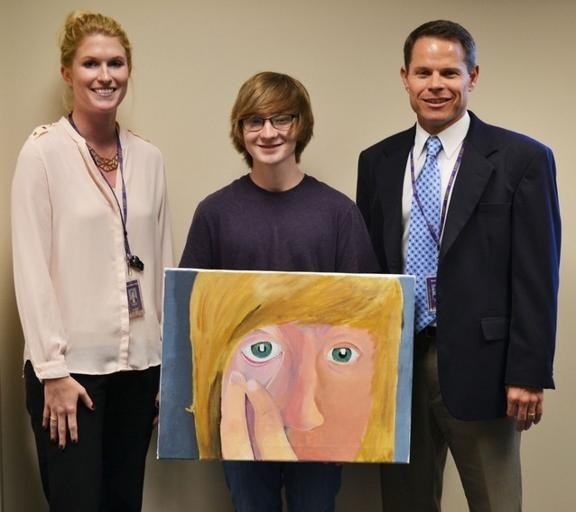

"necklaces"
[87,142,119,174]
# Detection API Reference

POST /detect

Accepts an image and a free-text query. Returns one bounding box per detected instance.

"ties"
[407,138,442,332]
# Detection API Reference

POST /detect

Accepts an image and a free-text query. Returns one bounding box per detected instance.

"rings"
[49,416,57,422]
[528,412,537,417]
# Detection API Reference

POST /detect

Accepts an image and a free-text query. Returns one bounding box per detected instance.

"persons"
[186,265,406,466]
[8,9,176,512]
[354,19,565,512]
[177,70,383,510]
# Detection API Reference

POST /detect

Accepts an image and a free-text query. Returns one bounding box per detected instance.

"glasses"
[241,113,299,131]
